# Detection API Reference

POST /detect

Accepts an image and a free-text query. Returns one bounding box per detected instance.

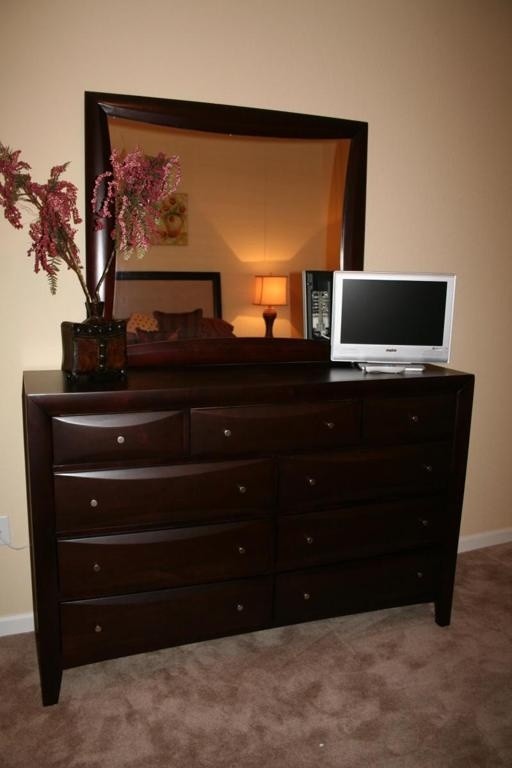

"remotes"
[365,365,405,374]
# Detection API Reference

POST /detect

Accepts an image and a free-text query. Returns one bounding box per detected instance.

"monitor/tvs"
[330,270,456,372]
[302,270,334,341]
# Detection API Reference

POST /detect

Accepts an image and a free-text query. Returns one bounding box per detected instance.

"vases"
[58,297,128,386]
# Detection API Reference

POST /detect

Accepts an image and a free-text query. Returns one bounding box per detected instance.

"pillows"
[152,308,204,340]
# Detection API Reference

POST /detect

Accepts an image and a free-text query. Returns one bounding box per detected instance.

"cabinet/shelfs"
[20,363,473,711]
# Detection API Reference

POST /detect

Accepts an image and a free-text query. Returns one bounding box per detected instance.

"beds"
[110,269,232,344]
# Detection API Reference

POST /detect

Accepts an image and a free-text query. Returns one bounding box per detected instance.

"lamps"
[249,275,288,337]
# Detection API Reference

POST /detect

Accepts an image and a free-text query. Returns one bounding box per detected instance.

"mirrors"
[84,90,369,368]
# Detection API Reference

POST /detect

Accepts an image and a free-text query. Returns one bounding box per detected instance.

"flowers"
[0,140,191,301]
